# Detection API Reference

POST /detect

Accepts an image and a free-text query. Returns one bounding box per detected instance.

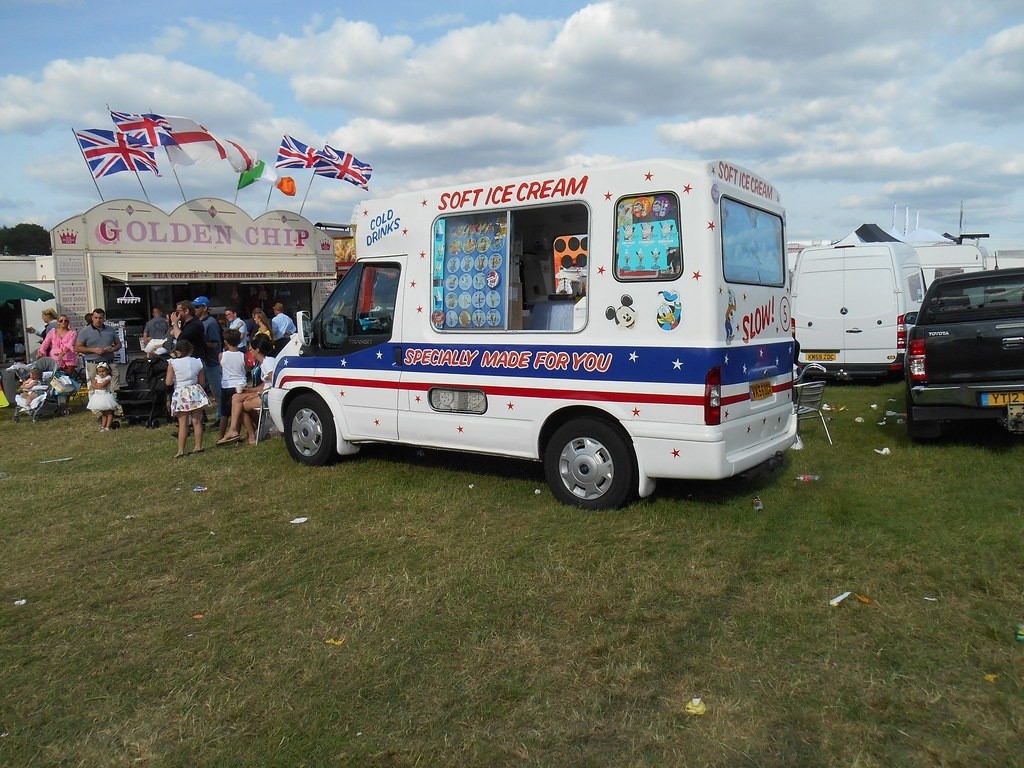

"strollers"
[6,353,80,422]
[111,356,174,430]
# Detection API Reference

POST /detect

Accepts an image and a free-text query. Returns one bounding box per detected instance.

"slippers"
[237,440,255,445]
[216,435,241,444]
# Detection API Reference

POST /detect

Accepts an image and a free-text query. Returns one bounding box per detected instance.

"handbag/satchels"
[51,377,75,393]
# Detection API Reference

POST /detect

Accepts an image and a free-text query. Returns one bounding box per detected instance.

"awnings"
[101,266,338,308]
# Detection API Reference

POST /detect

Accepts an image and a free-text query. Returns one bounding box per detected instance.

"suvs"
[903,267,1024,443]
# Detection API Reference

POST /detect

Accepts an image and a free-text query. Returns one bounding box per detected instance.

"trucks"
[790,242,928,383]
[267,158,799,509]
[153,285,227,323]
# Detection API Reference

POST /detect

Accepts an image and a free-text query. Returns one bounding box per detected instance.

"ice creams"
[349,204,359,235]
[623,222,672,240]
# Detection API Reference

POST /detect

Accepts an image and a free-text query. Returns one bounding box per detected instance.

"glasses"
[58,319,70,323]
[194,305,202,308]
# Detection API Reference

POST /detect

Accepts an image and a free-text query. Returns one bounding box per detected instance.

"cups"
[235,383,243,393]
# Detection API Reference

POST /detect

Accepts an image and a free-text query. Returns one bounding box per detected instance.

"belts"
[88,360,114,364]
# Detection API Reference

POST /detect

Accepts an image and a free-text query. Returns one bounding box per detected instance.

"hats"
[272,302,284,311]
[191,297,210,308]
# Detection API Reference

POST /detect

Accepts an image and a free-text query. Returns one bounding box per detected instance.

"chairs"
[938,295,971,310]
[253,390,269,445]
[792,381,834,445]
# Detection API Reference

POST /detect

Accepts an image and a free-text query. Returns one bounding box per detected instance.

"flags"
[157,112,259,173]
[110,109,183,152]
[235,154,297,196]
[274,134,328,170]
[74,129,163,179]
[312,143,373,192]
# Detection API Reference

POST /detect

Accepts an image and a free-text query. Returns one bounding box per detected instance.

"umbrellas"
[0,281,56,308]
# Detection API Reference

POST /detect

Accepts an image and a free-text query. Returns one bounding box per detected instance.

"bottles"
[752,495,763,512]
[794,474,820,481]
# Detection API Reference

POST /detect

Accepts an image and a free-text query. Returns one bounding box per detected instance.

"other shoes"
[212,421,221,428]
[99,427,109,433]
[173,431,191,437]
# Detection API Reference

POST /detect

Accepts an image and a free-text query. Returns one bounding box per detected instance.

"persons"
[19,369,41,408]
[73,308,125,424]
[86,361,118,432]
[135,296,316,436]
[217,335,276,445]
[25,309,59,344]
[36,314,78,412]
[166,340,212,458]
